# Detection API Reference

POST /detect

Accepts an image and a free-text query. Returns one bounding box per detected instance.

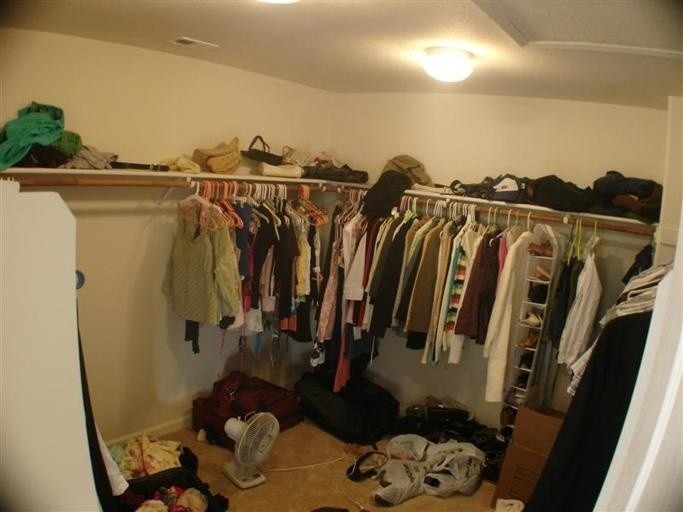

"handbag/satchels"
[192,371,401,452]
[382,154,435,188]
[192,135,369,184]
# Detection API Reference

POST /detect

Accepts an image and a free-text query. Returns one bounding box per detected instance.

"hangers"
[181,180,330,231]
[335,190,534,247]
[564,221,601,264]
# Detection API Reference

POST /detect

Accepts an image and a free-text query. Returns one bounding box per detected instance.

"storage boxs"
[491,408,565,512]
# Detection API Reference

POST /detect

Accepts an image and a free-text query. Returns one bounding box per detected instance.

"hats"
[493,173,521,201]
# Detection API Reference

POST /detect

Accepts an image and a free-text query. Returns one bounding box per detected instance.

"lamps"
[420,45,481,81]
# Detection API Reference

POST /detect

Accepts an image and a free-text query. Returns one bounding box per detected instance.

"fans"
[222,412,281,490]
